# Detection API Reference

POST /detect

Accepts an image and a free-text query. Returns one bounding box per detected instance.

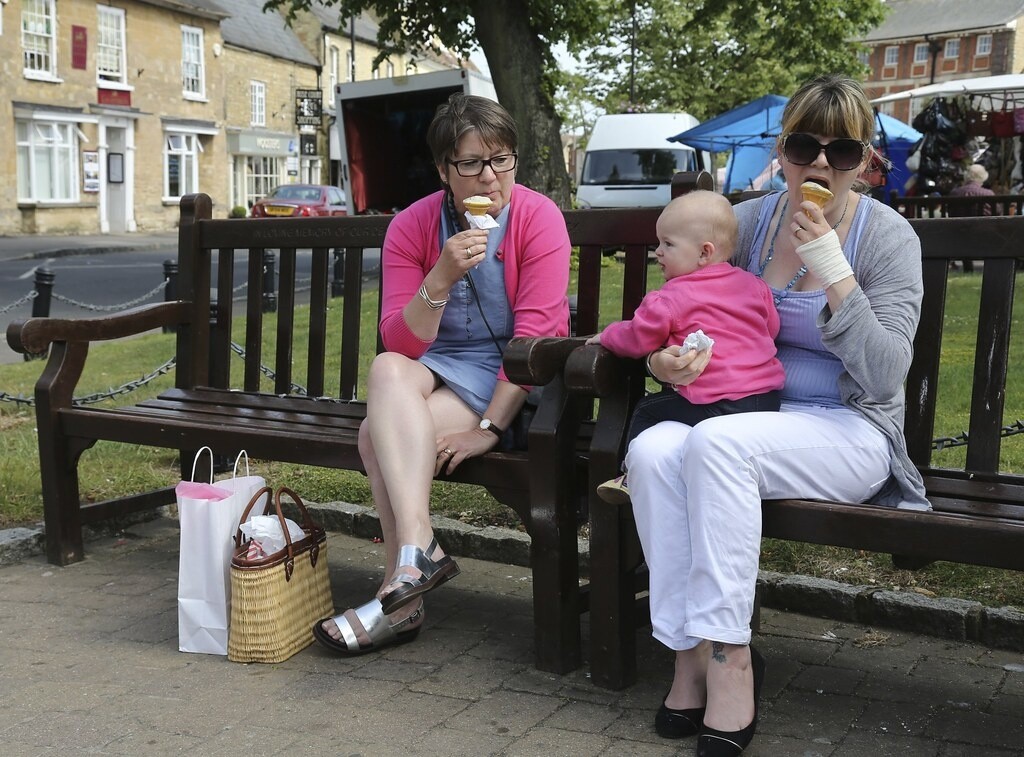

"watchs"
[479,419,504,439]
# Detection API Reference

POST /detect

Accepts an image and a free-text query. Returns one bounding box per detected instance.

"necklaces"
[756,194,849,308]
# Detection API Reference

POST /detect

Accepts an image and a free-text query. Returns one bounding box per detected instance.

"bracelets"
[417,284,451,310]
[646,347,674,383]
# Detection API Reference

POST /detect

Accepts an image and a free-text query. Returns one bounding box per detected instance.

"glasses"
[447,148,518,177]
[782,132,870,171]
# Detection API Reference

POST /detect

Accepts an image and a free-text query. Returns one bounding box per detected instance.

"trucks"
[575,111,713,215]
[333,66,502,219]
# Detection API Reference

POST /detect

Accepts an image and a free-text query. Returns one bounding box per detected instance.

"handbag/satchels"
[176,446,267,656]
[227,488,335,663]
[912,93,1024,195]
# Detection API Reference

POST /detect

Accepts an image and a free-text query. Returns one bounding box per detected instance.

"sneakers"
[596,473,630,505]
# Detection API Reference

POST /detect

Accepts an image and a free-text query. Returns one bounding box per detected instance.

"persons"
[922,165,1024,272]
[626,74,934,757]
[314,93,570,655]
[585,189,787,505]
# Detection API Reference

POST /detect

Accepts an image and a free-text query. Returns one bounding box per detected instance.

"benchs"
[6,171,712,675]
[889,188,1024,274]
[562,213,1024,693]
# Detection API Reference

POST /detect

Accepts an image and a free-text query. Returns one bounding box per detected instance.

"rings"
[444,449,453,458]
[793,227,803,237]
[466,248,472,259]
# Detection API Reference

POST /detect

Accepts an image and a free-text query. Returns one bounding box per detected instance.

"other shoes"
[697,644,765,756]
[655,682,707,738]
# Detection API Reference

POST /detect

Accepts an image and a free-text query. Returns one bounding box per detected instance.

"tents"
[667,94,924,207]
[868,74,1024,122]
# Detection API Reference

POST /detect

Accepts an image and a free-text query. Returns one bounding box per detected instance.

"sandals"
[381,537,461,614]
[312,590,424,653]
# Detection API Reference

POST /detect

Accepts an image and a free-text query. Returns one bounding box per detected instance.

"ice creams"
[463,194,494,230]
[800,181,835,221]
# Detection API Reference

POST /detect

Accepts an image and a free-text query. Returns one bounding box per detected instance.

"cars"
[247,184,347,218]
